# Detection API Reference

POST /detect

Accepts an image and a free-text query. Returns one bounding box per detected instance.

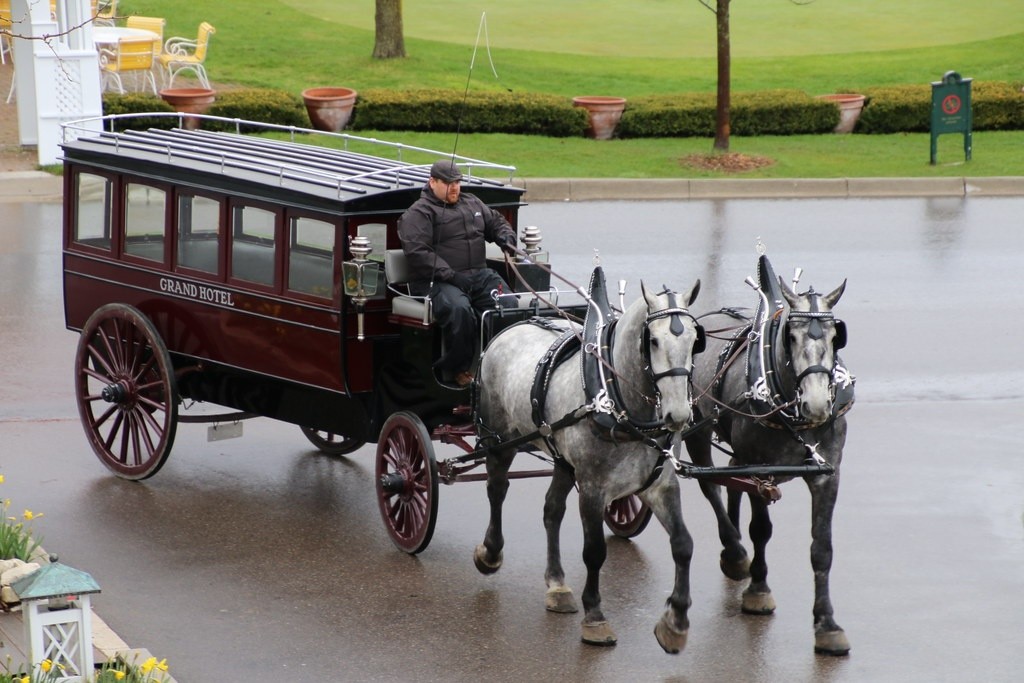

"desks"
[92,27,160,93]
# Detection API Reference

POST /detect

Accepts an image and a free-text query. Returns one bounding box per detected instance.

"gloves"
[497,235,515,256]
[450,274,472,294]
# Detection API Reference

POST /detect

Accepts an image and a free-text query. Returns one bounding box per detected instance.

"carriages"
[54,109,857,661]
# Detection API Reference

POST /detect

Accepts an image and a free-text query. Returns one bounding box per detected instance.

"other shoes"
[454,371,474,385]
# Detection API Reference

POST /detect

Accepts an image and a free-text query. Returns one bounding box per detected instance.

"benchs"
[105,238,385,298]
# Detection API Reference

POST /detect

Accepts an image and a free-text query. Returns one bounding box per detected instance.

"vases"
[301,87,357,133]
[572,95,626,140]
[814,93,865,135]
[160,87,216,130]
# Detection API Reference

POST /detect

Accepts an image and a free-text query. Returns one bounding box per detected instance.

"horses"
[470,256,863,658]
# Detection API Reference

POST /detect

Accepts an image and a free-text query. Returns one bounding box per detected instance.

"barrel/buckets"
[301,87,357,132]
[160,89,217,129]
[816,94,867,133]
[573,96,627,139]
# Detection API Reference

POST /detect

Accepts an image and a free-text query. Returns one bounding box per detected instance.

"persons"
[397,159,519,387]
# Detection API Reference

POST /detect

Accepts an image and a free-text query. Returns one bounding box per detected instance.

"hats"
[431,159,463,182]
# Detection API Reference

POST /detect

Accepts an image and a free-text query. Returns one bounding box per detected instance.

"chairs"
[157,21,216,90]
[0,0,117,65]
[127,16,167,94]
[98,36,160,96]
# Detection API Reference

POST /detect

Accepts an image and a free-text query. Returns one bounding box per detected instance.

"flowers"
[0,651,172,683]
[0,474,47,563]
